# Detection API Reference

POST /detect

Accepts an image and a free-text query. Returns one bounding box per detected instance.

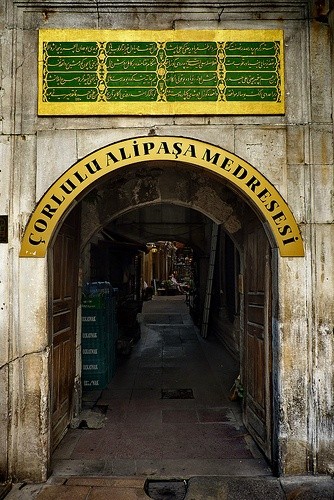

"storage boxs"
[82,291,115,390]
